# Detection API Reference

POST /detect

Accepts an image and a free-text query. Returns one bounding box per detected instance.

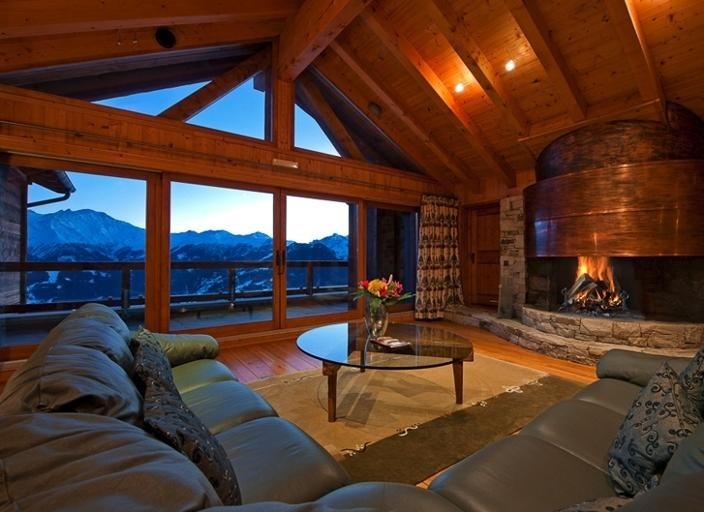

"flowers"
[350,276,417,338]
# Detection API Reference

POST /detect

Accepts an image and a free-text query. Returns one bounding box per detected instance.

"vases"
[362,311,389,340]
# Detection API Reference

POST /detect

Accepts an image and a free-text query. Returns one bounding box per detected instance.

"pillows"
[607,344,704,500]
[130,325,244,505]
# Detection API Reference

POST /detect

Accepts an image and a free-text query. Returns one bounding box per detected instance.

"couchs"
[0,297,703,512]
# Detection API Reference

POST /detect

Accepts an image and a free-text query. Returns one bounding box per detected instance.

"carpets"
[244,338,589,485]
